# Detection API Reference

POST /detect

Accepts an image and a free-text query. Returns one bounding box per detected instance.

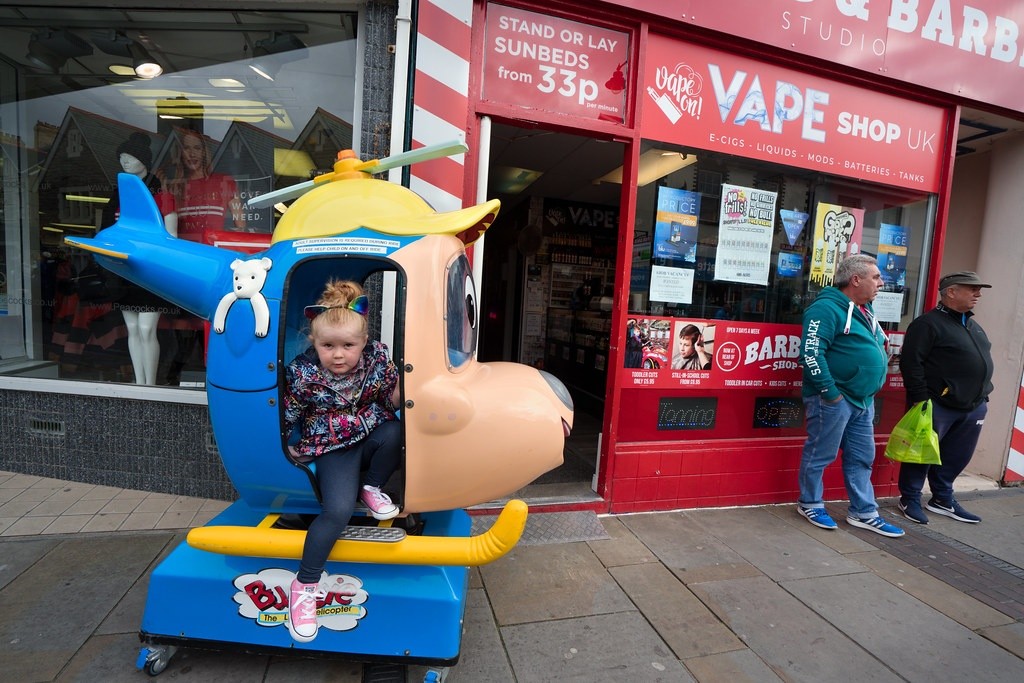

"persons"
[156,126,246,244]
[624,318,713,371]
[283,281,405,642]
[898,270,994,525]
[797,255,905,537]
[109,133,178,385]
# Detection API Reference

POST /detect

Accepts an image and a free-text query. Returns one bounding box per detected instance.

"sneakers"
[926,496,981,522]
[898,497,929,524]
[797,505,838,529]
[847,515,905,537]
[289,578,319,643]
[358,485,399,520]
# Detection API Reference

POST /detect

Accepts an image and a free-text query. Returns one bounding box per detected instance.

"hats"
[939,271,992,288]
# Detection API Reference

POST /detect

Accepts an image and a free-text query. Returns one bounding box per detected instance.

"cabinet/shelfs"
[548,263,616,309]
[690,280,768,323]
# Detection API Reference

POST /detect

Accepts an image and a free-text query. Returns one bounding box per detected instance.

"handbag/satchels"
[884,398,942,465]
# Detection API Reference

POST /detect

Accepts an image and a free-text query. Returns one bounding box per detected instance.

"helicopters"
[60,141,575,566]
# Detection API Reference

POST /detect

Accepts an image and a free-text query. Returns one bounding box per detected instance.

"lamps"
[91,28,163,79]
[249,30,309,81]
[605,60,627,95]
[26,26,93,72]
